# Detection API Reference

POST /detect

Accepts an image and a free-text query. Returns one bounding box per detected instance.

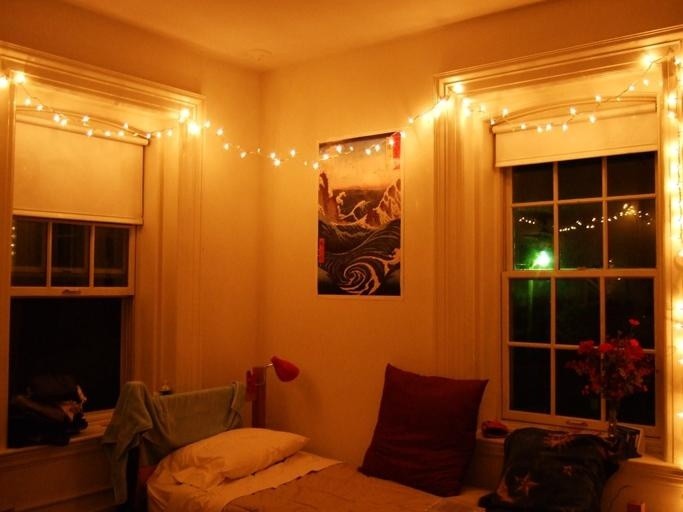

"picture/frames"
[316,130,402,297]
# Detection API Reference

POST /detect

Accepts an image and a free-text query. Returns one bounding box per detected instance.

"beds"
[125,366,495,512]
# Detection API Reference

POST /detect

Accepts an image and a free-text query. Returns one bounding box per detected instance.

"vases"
[607,411,617,439]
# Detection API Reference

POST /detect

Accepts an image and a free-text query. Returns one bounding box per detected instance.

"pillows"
[358,363,490,499]
[478,428,626,512]
[155,427,308,490]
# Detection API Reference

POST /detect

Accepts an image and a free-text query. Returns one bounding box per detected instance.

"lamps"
[246,356,299,400]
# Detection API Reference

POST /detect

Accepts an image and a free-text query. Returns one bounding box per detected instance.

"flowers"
[567,318,651,408]
